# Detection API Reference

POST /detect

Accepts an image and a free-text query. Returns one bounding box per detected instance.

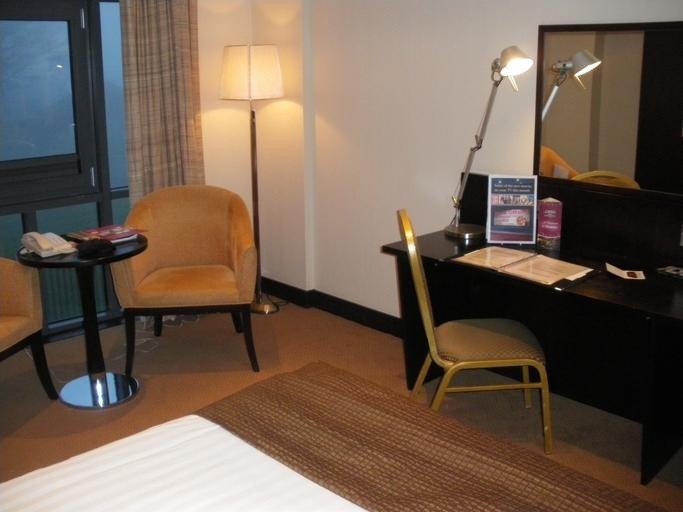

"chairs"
[110,185,260,375]
[1,257,60,400]
[395,209,553,457]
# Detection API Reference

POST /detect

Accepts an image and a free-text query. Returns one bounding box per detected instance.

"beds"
[0,360,666,511]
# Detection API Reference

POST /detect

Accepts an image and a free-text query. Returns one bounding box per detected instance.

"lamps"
[443,46,533,240]
[541,49,603,121]
[220,43,286,315]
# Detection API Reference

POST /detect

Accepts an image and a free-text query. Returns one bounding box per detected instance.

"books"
[440,243,598,292]
[67,219,148,243]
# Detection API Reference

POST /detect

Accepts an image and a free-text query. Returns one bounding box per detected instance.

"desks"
[382,222,682,484]
[18,231,148,410]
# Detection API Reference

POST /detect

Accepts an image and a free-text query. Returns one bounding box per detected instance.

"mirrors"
[534,22,647,198]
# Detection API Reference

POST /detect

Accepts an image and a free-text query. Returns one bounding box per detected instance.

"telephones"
[20,232,78,257]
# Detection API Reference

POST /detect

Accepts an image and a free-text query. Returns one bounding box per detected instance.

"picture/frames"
[486,174,538,245]
